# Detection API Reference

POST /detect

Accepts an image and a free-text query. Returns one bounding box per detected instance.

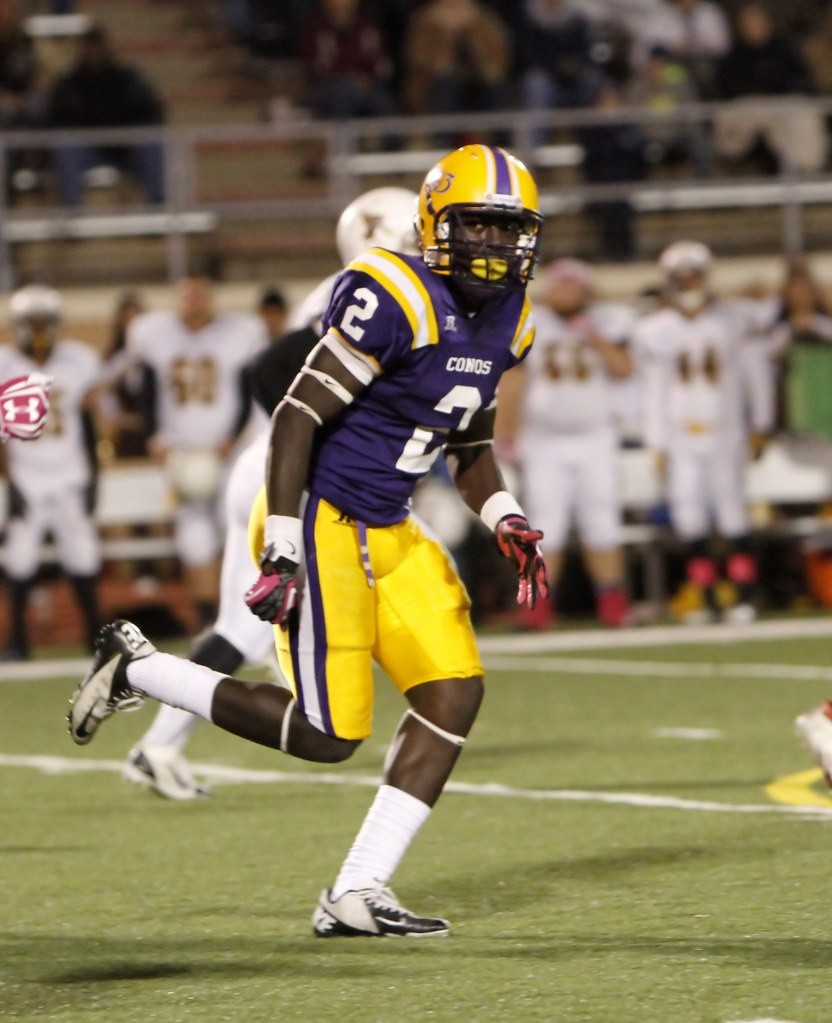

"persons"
[496,252,637,629]
[0,285,112,662]
[0,0,827,189]
[794,694,832,789]
[94,290,201,644]
[746,256,832,615]
[125,187,425,801]
[631,236,776,626]
[125,257,270,623]
[250,290,288,341]
[64,142,552,937]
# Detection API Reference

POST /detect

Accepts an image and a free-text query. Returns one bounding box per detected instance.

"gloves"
[490,513,551,609]
[243,544,299,630]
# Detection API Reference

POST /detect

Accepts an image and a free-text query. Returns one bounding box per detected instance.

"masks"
[673,288,708,316]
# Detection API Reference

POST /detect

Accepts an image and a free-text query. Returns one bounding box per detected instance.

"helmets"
[546,255,592,288]
[331,182,427,265]
[10,280,64,323]
[652,236,710,270]
[414,144,544,308]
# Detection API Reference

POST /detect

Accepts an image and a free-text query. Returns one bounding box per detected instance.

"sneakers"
[68,620,157,745]
[117,732,202,802]
[312,885,454,938]
